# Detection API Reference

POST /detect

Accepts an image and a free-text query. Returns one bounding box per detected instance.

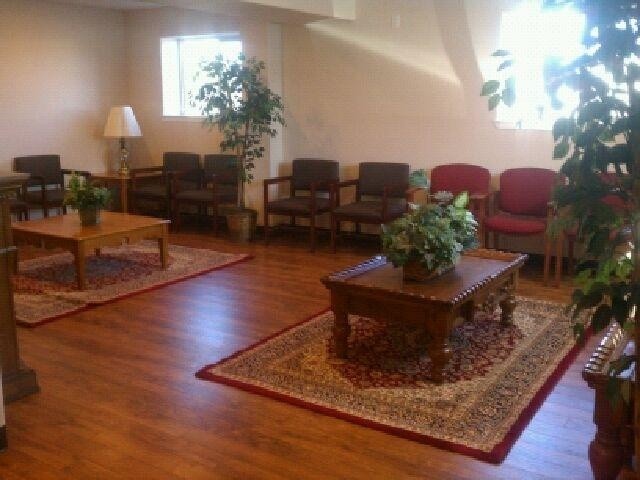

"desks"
[580,303,639,479]
[11,208,172,291]
[89,171,168,214]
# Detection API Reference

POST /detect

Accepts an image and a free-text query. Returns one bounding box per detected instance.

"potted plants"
[59,170,112,227]
[190,52,288,240]
[379,190,479,282]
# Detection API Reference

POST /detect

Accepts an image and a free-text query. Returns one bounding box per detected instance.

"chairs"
[0,174,28,219]
[563,171,640,283]
[331,161,410,250]
[409,163,495,250]
[126,151,238,237]
[13,153,92,221]
[264,159,341,253]
[482,168,560,285]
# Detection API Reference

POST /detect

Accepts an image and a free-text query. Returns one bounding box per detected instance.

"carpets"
[194,294,605,466]
[12,239,256,331]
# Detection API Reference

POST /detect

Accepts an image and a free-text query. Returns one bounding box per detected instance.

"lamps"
[103,104,143,176]
